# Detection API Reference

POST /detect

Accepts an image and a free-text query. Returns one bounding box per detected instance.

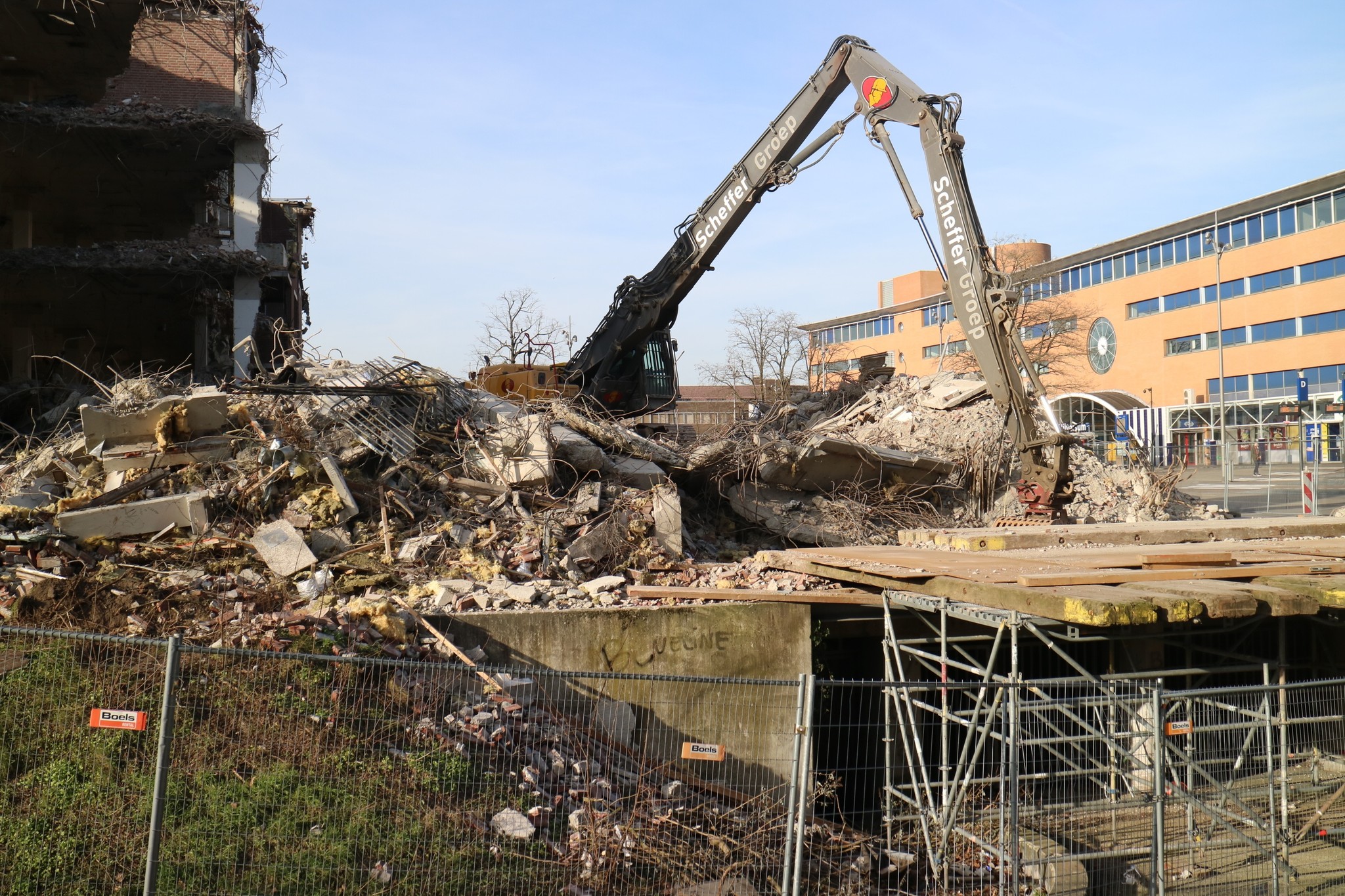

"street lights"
[561,316,578,361]
[816,331,830,396]
[931,297,949,372]
[729,358,738,433]
[770,380,780,407]
[1143,387,1153,470]
[1204,232,1233,478]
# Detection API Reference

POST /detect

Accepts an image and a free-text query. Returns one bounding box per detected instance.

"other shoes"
[1257,474,1262,477]
[1253,474,1256,476]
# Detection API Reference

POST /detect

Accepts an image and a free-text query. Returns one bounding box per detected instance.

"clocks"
[1097,336,1108,355]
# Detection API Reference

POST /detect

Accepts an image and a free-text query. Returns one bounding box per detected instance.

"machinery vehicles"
[457,34,1083,527]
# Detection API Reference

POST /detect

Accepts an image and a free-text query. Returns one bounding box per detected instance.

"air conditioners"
[1184,389,1194,404]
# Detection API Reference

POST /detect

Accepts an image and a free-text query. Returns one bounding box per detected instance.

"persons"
[1252,440,1262,476]
